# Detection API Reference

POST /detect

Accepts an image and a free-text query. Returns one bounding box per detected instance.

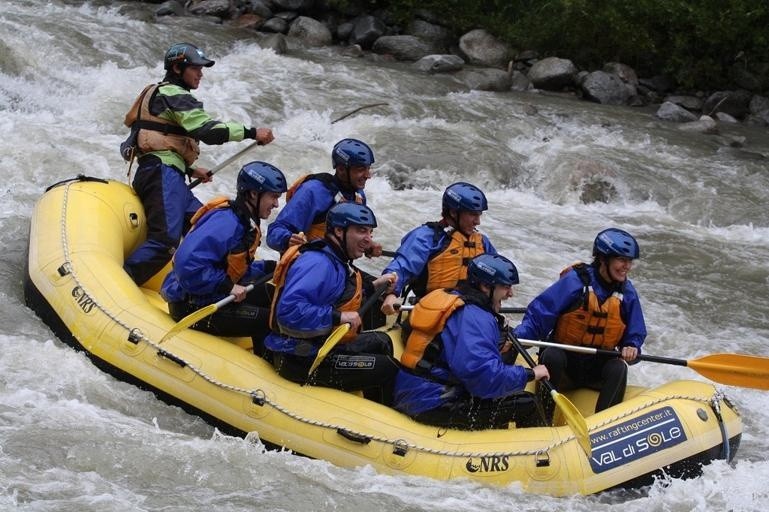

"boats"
[23,178,743,497]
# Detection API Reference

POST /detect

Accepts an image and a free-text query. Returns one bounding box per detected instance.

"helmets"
[164,42,215,70]
[332,138,375,169]
[592,227,640,260]
[467,253,520,287]
[326,201,377,234]
[236,161,288,195]
[442,182,488,218]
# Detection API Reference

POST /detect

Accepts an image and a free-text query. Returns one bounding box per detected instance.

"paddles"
[505,326,593,458]
[160,270,275,344]
[309,272,398,376]
[518,337,765,390]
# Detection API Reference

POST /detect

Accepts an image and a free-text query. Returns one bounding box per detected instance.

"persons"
[394,253,551,428]
[266,138,383,258]
[379,182,498,316]
[119,43,274,287]
[501,228,648,428]
[159,160,289,358]
[264,202,400,406]
